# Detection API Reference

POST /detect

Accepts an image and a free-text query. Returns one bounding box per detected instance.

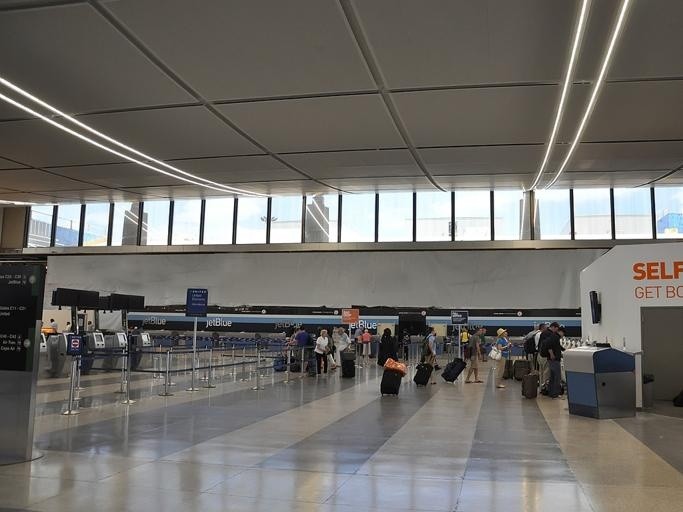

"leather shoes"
[496,384,504,388]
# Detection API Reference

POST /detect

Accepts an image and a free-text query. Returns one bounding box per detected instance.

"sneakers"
[553,395,565,400]
[465,380,483,383]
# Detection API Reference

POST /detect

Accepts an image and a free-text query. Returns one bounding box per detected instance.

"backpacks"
[421,335,433,355]
[524,330,539,353]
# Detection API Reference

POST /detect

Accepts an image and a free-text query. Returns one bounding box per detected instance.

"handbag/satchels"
[489,346,502,361]
[328,336,333,347]
[383,358,406,377]
[343,351,356,360]
[464,345,472,358]
[542,379,564,396]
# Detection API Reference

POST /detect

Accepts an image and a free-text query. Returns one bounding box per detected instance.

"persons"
[287,326,371,375]
[379,328,513,389]
[525,322,565,399]
[48,318,94,336]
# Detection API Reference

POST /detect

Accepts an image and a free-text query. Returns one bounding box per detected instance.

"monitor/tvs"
[589,291,600,324]
[39,334,149,344]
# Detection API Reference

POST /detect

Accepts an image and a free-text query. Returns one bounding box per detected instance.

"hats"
[497,328,506,337]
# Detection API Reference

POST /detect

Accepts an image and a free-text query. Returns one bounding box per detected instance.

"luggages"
[273,354,301,373]
[414,355,436,386]
[442,357,467,383]
[503,349,539,398]
[380,369,402,396]
[342,349,355,376]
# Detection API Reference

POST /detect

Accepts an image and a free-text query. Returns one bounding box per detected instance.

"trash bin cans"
[643,374,654,406]
